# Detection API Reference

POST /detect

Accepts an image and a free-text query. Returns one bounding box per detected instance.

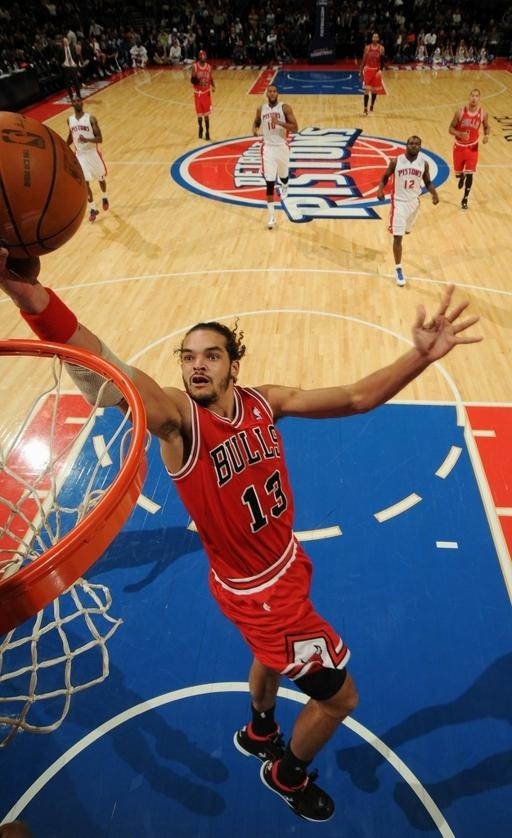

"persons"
[252,84,298,229]
[65,96,109,223]
[190,49,217,138]
[125,1,512,67]
[449,89,490,209]
[377,135,439,286]
[1,1,125,85]
[56,37,83,100]
[358,32,389,116]
[0,244,485,824]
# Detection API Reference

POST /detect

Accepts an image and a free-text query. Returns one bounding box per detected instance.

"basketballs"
[0,112,86,258]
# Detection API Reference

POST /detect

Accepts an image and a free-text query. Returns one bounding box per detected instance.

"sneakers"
[280,186,288,200]
[458,177,468,210]
[396,267,407,286]
[233,720,286,763]
[89,209,99,221]
[102,198,109,211]
[260,760,336,822]
[268,216,276,230]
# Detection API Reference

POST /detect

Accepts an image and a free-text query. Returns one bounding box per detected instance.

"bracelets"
[20,288,78,344]
